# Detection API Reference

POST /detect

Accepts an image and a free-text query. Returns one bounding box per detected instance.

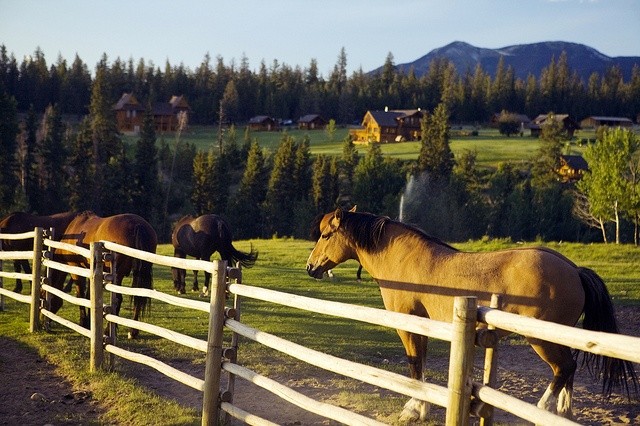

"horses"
[169,213,259,298]
[0,211,89,294]
[304,203,636,425]
[42,210,157,339]
